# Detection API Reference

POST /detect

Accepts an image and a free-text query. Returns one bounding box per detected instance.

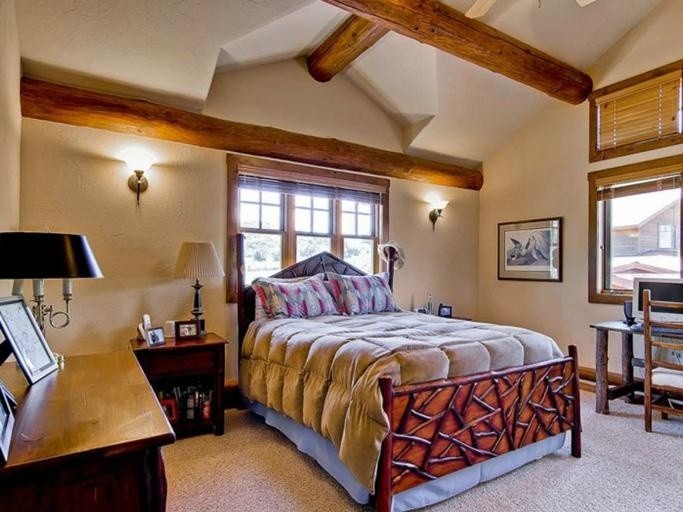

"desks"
[588,320,683,415]
[1,349,176,512]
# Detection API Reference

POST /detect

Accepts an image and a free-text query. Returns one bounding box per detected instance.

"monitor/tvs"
[632,277,683,322]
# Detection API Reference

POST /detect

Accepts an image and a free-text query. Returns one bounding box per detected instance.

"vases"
[145,326,166,347]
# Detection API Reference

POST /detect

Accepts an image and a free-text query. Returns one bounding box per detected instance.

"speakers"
[623,301,637,327]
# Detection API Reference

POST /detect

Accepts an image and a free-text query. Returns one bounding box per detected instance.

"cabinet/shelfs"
[128,332,229,439]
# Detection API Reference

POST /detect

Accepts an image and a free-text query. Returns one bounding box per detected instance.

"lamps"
[173,242,226,336]
[0,232,106,368]
[429,200,448,230]
[123,159,154,206]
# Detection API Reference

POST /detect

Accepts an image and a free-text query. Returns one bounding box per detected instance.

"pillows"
[251,273,347,319]
[325,272,405,314]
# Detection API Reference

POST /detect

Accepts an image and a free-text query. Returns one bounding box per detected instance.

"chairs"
[643,289,683,431]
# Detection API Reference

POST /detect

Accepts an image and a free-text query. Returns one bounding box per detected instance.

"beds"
[235,234,581,512]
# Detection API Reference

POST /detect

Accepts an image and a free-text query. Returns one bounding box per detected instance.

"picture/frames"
[497,217,562,281]
[175,321,200,341]
[1,390,17,463]
[0,295,60,384]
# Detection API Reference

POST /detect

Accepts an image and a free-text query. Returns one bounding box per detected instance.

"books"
[156,385,215,420]
